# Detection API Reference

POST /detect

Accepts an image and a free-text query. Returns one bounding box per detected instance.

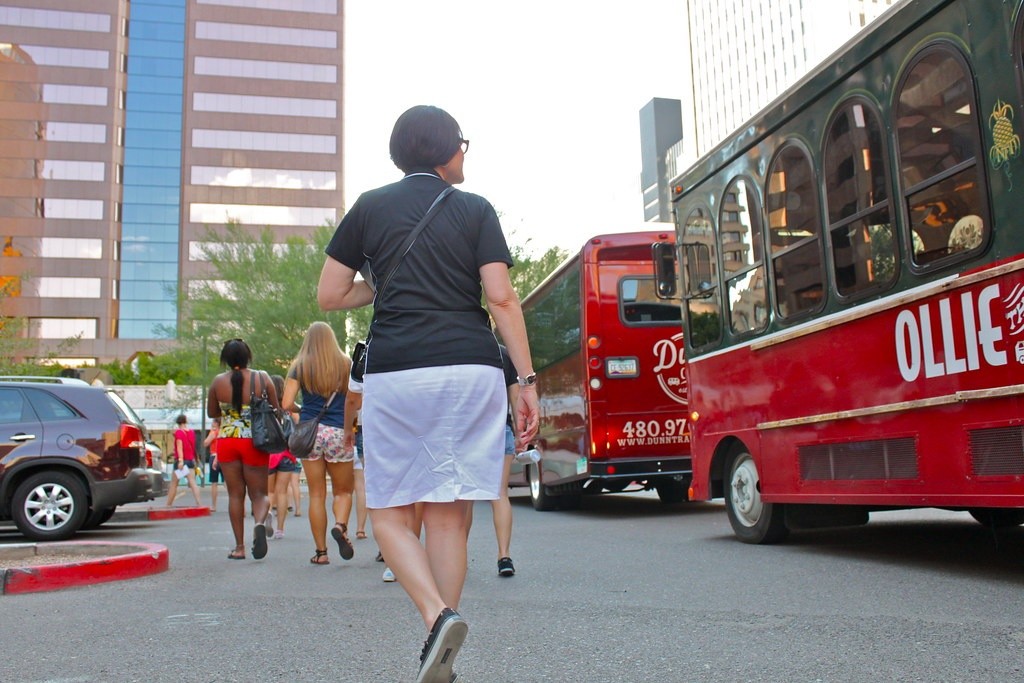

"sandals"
[356,531,367,539]
[375,550,382,561]
[331,522,354,560]
[310,549,330,565]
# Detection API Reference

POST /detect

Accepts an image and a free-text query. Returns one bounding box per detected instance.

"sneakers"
[415,606,468,682]
[498,557,515,576]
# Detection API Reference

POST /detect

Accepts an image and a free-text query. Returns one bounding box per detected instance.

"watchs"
[517,372,537,387]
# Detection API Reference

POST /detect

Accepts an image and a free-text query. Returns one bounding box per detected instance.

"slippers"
[251,523,268,559]
[227,549,246,560]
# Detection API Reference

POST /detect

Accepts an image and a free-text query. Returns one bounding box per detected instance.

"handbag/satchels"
[250,369,292,453]
[287,418,320,457]
[350,341,370,384]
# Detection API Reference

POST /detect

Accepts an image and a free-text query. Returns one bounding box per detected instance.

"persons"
[282,321,365,564]
[203,338,301,559]
[166,414,204,507]
[315,105,541,683]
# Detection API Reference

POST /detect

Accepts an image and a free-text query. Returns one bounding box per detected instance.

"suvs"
[0,376,171,542]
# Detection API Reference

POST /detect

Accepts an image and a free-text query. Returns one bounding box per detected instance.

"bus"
[493,230,699,511]
[651,0,1024,543]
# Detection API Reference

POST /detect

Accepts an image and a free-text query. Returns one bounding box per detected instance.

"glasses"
[459,137,469,154]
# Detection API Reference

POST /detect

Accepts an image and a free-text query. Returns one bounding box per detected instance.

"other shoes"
[265,513,274,538]
[288,507,293,512]
[383,567,395,581]
[275,530,284,539]
[294,511,301,516]
